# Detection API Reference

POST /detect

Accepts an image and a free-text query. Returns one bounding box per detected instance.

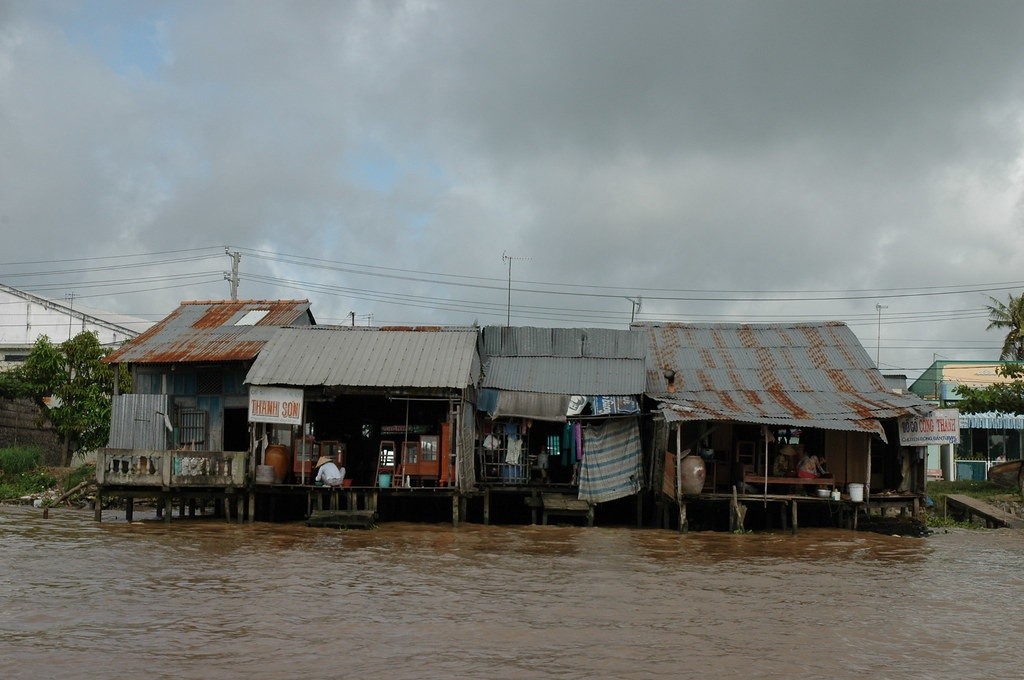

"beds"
[743,470,836,497]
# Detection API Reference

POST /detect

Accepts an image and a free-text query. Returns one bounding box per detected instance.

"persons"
[315,457,346,487]
[996,452,1007,465]
[773,445,828,479]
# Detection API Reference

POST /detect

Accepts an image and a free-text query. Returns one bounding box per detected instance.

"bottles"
[538,447,548,469]
[406,475,410,487]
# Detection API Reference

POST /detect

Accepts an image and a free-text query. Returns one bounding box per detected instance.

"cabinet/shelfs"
[294,423,457,488]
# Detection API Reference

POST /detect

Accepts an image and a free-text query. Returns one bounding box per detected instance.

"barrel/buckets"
[343,479,352,486]
[832,488,840,500]
[379,470,391,487]
[699,449,714,459]
[256,465,273,483]
[849,483,864,502]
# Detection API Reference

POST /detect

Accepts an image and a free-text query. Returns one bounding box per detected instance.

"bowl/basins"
[818,489,831,497]
[315,482,323,486]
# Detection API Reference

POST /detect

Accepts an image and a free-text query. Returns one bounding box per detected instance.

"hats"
[315,456,333,468]
[779,445,797,456]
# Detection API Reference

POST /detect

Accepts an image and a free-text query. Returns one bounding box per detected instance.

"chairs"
[392,467,406,487]
[736,441,755,473]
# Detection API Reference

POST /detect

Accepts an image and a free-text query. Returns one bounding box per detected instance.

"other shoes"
[322,484,331,488]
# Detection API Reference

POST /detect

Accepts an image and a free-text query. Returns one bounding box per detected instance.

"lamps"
[663,369,677,399]
